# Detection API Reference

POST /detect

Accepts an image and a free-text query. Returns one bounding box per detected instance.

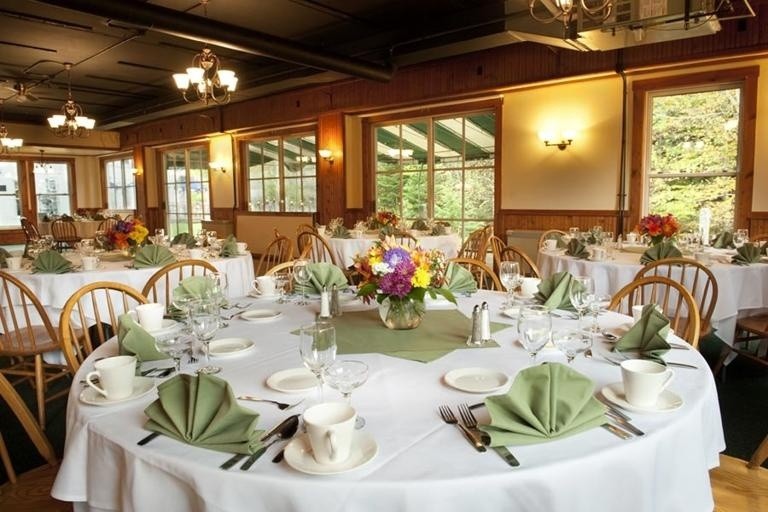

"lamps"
[318,149,335,165]
[1,62,96,157]
[170,0,242,108]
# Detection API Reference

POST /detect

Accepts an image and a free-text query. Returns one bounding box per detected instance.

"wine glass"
[273,259,313,307]
[297,321,370,430]
[498,261,612,370]
[559,227,749,250]
[327,216,366,232]
[152,272,227,376]
[25,228,226,262]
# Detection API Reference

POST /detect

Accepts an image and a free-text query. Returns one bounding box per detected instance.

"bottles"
[317,282,342,318]
[470,301,490,345]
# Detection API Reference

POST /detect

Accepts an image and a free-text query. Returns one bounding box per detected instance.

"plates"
[202,335,255,357]
[265,367,326,392]
[247,291,277,300]
[599,382,683,416]
[239,309,282,322]
[154,318,177,335]
[444,367,510,393]
[78,376,156,406]
[282,432,379,476]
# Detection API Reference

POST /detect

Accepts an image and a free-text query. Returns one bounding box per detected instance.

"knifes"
[219,416,297,470]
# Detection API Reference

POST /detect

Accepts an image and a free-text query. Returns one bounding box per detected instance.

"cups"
[620,359,673,406]
[81,257,100,272]
[127,303,167,333]
[695,253,705,264]
[250,275,275,296]
[5,257,22,272]
[410,229,416,237]
[234,242,247,254]
[542,239,557,251]
[317,225,326,236]
[592,249,605,260]
[301,401,358,466]
[632,306,665,329]
[86,355,137,400]
[444,227,450,235]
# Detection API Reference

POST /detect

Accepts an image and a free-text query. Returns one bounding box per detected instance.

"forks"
[456,403,520,467]
[79,368,156,386]
[220,311,245,321]
[602,355,697,370]
[439,402,486,453]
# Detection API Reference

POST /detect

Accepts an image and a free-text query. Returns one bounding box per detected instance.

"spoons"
[242,415,299,471]
[152,368,172,378]
[601,331,690,350]
[237,397,290,411]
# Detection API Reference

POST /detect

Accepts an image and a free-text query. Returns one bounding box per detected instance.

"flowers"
[633,210,679,246]
[371,210,396,230]
